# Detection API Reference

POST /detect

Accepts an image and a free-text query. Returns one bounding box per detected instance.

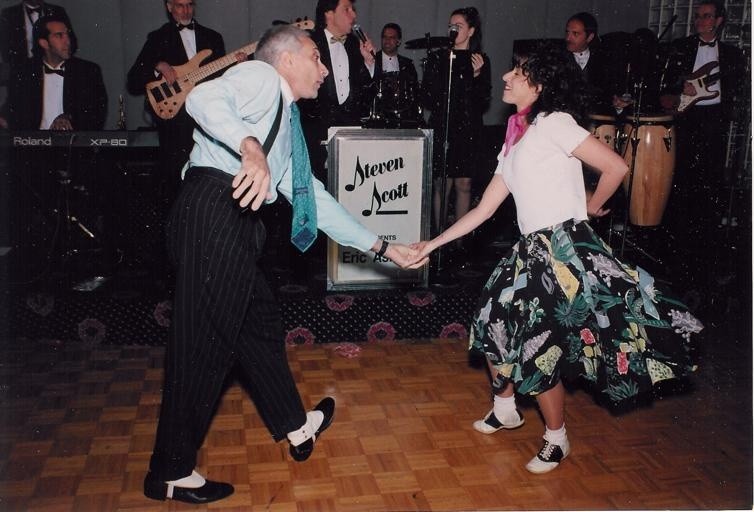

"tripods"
[599,49,663,270]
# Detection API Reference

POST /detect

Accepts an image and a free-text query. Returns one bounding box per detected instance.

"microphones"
[449,26,458,48]
[352,24,377,60]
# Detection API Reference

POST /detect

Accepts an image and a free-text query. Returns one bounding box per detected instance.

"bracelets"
[377,238,390,257]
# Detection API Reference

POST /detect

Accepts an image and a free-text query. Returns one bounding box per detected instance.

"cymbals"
[405,37,450,51]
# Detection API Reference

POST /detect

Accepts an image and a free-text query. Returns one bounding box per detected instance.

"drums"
[621,116,678,227]
[580,115,618,200]
[357,78,418,128]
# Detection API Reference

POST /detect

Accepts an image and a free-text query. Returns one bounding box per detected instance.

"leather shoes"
[144,478,234,504]
[290,397,334,461]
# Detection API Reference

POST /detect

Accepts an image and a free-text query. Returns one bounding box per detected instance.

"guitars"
[661,59,750,113]
[144,18,316,120]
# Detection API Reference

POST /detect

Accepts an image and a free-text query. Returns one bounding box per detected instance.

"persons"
[409,37,711,474]
[552,10,630,119]
[290,1,493,269]
[0,17,108,134]
[142,22,430,504]
[125,0,251,131]
[657,1,746,220]
[0,1,78,88]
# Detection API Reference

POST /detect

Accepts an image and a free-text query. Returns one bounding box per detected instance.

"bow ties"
[699,40,717,48]
[25,5,42,14]
[331,35,348,45]
[176,21,193,30]
[45,66,63,77]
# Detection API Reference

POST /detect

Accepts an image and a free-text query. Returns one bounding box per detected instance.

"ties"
[290,101,320,255]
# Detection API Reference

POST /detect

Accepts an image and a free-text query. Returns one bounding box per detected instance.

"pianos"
[1,129,159,152]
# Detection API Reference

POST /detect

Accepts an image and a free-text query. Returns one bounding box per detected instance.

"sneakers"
[474,408,525,434]
[526,437,570,473]
